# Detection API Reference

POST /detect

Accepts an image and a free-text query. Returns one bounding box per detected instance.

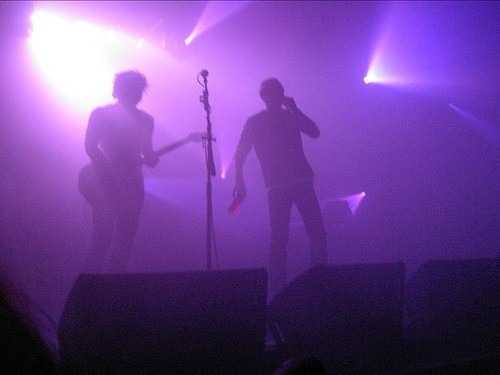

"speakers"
[403,259,500,343]
[59,267,268,365]
[269,264,404,356]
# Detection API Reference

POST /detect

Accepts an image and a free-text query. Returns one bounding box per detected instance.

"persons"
[81,70,161,272]
[273,356,327,375]
[232,77,329,292]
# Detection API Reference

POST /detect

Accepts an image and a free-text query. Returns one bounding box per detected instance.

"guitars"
[78,130,204,208]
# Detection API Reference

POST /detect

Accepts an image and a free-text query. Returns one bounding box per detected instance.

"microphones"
[280,98,295,116]
[200,69,209,83]
